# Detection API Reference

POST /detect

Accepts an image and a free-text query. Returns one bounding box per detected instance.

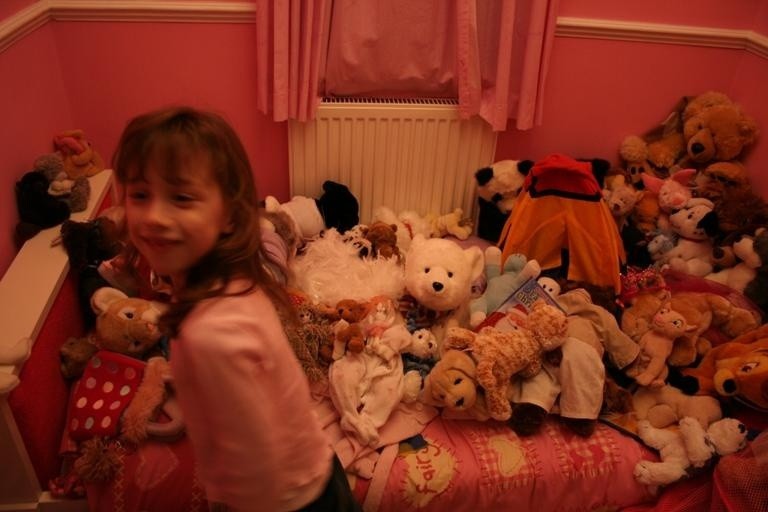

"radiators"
[286,94,499,235]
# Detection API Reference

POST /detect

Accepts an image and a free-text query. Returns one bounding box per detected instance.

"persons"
[108,105,363,512]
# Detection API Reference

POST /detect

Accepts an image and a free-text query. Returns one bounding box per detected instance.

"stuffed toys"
[13,86,768,491]
[11,130,101,257]
[262,181,567,445]
[472,86,768,490]
[49,245,183,491]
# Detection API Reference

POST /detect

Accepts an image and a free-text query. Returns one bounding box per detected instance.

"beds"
[0,169,768,512]
